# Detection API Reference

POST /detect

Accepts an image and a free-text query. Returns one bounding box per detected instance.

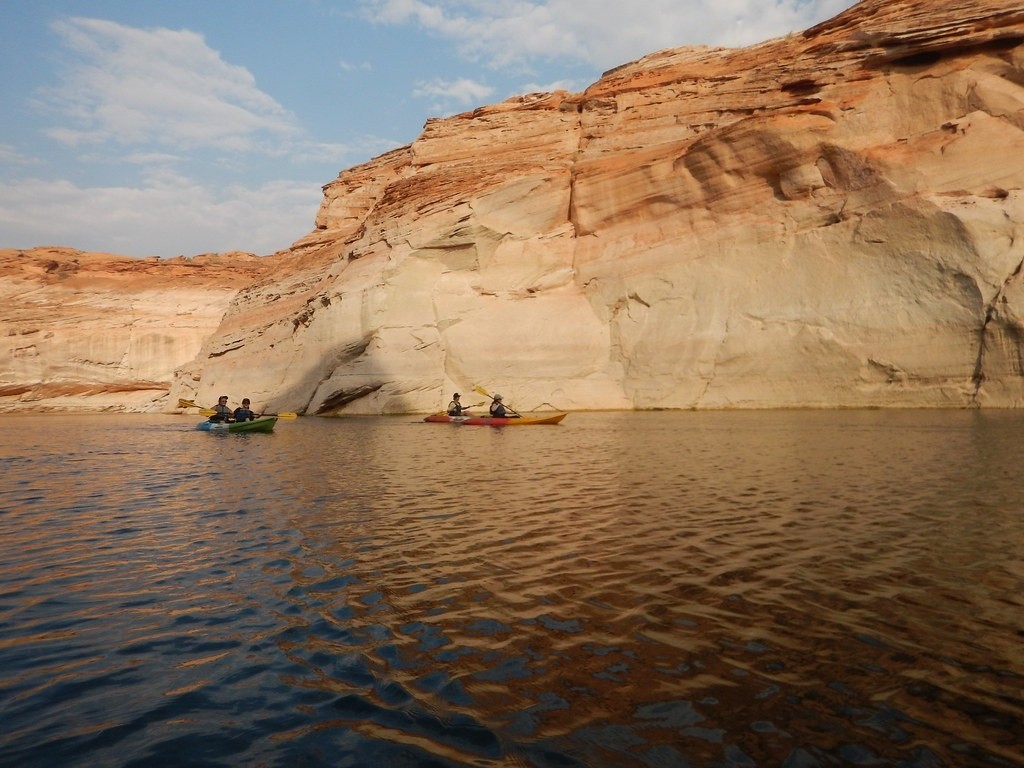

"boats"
[195,415,278,431]
[422,410,569,426]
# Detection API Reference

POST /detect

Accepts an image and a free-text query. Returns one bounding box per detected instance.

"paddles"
[199,409,297,421]
[476,386,523,417]
[436,401,486,416]
[178,399,206,409]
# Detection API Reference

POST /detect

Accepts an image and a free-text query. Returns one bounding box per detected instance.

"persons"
[489,394,520,419]
[228,398,262,422]
[206,396,232,423]
[447,393,474,416]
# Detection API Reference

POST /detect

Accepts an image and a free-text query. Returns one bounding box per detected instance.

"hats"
[218,395,228,399]
[241,398,250,404]
[494,394,504,400]
[453,393,461,400]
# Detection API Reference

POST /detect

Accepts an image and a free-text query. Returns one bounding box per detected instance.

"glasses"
[498,398,502,401]
[222,400,228,403]
